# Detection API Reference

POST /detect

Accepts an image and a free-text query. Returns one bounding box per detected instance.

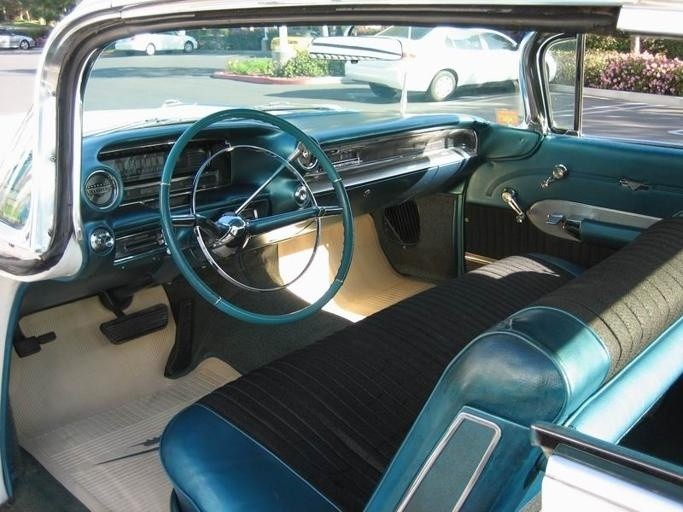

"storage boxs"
[161,213,683,512]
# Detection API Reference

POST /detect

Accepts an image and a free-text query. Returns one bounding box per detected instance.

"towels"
[261,39,268,51]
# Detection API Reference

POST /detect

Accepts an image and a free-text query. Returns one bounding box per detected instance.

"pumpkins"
[309,53,373,60]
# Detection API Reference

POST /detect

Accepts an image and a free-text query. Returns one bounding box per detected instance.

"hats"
[345,24,556,101]
[0,26,35,50]
[114,30,196,55]
